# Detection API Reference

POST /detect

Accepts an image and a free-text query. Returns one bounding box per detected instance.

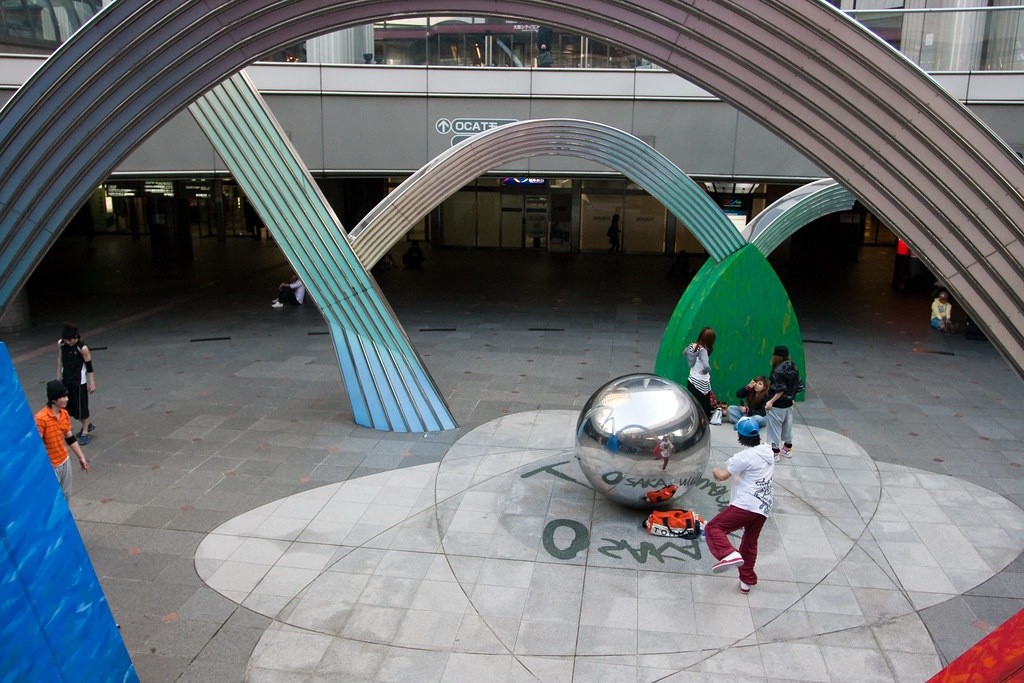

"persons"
[659,433,674,472]
[271,271,306,308]
[402,240,426,270]
[33,379,89,505]
[243,195,265,241]
[705,416,775,594]
[56,325,96,446]
[764,346,805,462]
[537,25,554,67]
[727,376,770,427]
[676,251,689,283]
[606,214,621,255]
[682,327,716,424]
[930,291,959,334]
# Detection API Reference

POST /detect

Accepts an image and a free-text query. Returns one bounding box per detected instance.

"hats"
[540,44,546,49]
[46,379,68,402]
[61,322,81,339]
[772,346,789,357]
[736,416,760,437]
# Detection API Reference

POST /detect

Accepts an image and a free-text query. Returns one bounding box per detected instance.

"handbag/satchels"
[642,485,677,504]
[642,509,704,539]
[709,407,723,424]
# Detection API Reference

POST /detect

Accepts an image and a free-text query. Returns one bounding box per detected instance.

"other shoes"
[721,404,727,416]
[271,302,284,308]
[772,452,781,462]
[272,298,279,303]
[778,446,793,457]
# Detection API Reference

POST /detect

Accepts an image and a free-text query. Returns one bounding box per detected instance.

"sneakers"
[739,581,751,594]
[78,433,93,446]
[711,550,745,574]
[75,423,95,438]
